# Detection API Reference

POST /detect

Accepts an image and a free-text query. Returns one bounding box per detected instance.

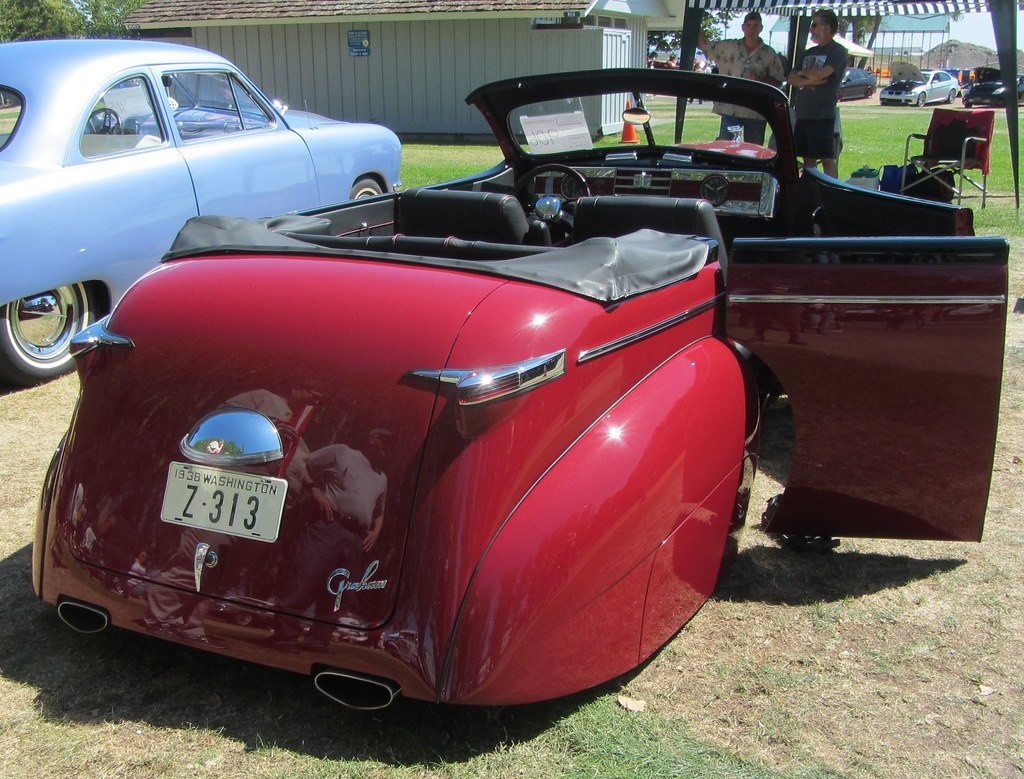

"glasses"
[810,22,828,28]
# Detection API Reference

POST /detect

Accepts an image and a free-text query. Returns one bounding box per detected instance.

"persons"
[788,9,848,179]
[738,280,942,346]
[697,12,784,146]
[63,375,402,649]
[647,50,718,105]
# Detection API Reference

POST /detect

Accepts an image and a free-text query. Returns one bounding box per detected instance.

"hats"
[365,427,400,438]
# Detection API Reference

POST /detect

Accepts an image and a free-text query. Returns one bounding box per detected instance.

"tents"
[676,0,1024,225]
[806,34,874,74]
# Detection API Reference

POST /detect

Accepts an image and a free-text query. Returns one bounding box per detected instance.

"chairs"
[573,195,727,287]
[904,108,996,208]
[395,189,551,247]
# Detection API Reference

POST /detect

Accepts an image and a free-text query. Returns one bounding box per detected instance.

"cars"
[2,37,406,386]
[30,70,1011,720]
[832,45,1023,110]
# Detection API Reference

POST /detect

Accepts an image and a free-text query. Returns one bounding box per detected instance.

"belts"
[327,510,369,536]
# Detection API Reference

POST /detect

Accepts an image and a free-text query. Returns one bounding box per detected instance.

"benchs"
[292,227,568,259]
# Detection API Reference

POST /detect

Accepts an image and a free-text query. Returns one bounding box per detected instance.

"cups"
[863,165,869,172]
